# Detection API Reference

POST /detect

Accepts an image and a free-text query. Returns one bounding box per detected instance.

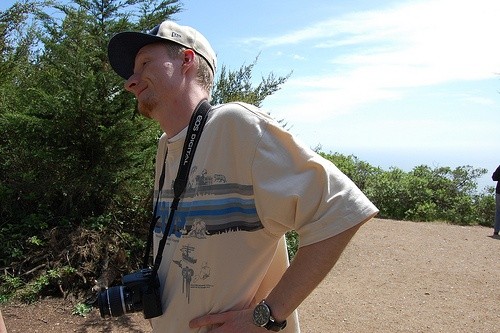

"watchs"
[252,300,287,331]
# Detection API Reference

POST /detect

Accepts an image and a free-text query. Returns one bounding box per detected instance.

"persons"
[109,20,379,333]
[488,163,500,237]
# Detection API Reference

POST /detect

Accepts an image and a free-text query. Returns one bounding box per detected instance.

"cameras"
[98,267,162,319]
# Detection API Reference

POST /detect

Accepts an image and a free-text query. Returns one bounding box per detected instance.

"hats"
[107,21,217,80]
